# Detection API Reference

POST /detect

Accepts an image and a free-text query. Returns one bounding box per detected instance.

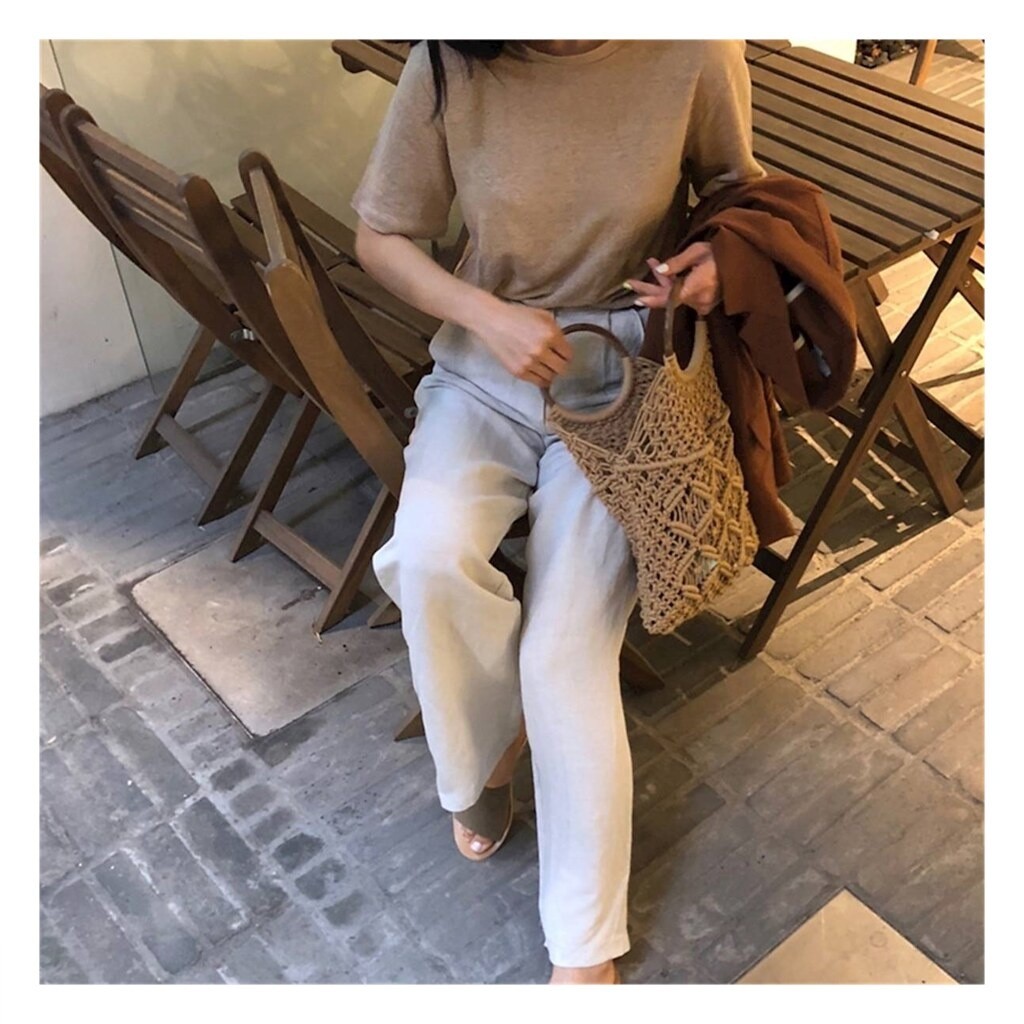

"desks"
[334,39,985,664]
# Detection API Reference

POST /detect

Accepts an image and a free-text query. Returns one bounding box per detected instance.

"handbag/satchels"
[540,278,759,636]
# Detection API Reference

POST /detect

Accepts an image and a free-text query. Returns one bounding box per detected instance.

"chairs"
[39,79,666,742]
[860,40,986,492]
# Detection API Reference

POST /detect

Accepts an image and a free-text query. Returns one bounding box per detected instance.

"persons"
[349,39,769,983]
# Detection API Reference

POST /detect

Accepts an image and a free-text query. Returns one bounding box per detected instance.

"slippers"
[452,737,529,860]
